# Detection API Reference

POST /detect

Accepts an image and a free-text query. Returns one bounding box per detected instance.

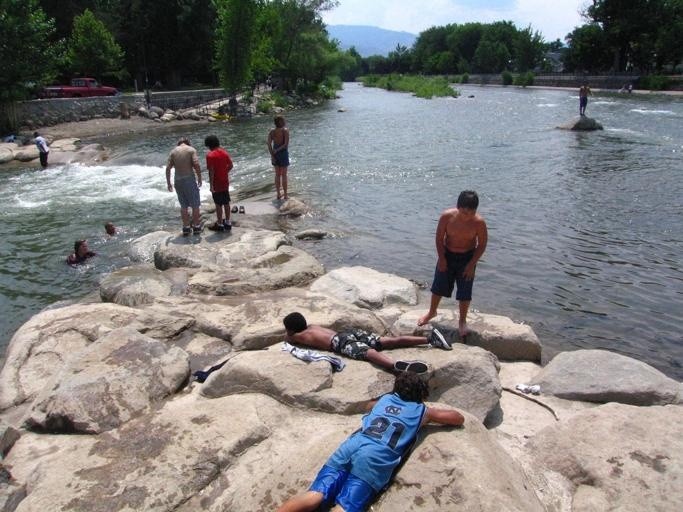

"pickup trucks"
[37,78,119,98]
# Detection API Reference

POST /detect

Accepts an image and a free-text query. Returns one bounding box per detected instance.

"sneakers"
[394,360,429,375]
[431,328,452,350]
[231,206,238,213]
[239,206,245,213]
[183,224,205,235]
[207,219,231,231]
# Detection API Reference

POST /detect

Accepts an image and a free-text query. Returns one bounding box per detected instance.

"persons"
[204,134,233,231]
[66,239,97,266]
[283,312,452,375]
[418,189,487,337]
[274,371,464,512]
[578,80,592,115]
[104,222,115,236]
[267,116,288,199]
[33,131,50,168]
[165,137,202,236]
